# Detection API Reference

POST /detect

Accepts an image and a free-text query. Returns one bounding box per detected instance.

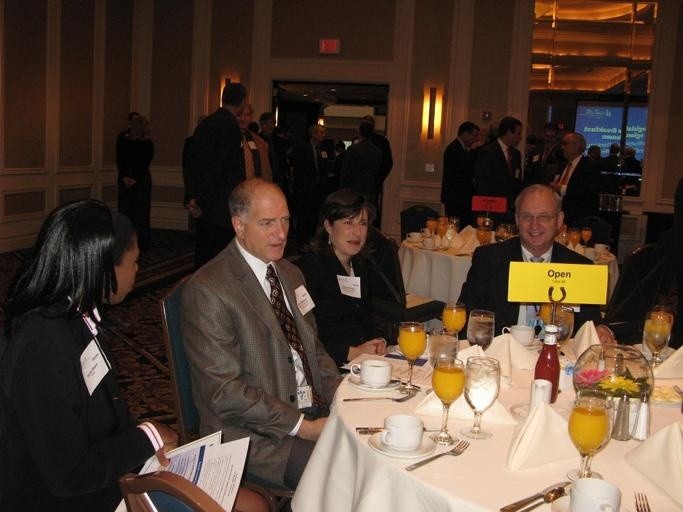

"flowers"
[575,369,653,399]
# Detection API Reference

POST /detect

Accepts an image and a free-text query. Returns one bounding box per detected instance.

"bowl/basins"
[573,345,654,403]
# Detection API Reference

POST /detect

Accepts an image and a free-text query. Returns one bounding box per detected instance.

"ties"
[560,162,574,186]
[262,262,318,407]
[527,254,544,333]
[505,148,512,171]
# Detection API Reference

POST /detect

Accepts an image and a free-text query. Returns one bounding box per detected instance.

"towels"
[506,402,581,472]
[571,319,603,363]
[455,233,481,256]
[625,422,683,512]
[454,344,487,378]
[646,342,683,380]
[484,333,538,378]
[459,223,477,234]
[414,380,520,426]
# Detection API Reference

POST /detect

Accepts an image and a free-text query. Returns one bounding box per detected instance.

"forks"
[635,492,651,512]
[344,392,415,402]
[356,427,448,432]
[405,440,471,471]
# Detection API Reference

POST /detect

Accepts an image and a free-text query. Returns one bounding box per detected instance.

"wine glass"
[566,390,613,480]
[644,305,676,369]
[555,224,610,260]
[407,216,511,256]
[398,302,500,445]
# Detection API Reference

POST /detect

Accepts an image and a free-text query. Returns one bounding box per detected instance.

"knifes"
[500,481,572,512]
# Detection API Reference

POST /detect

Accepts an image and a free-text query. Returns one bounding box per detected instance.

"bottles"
[612,392,650,441]
[598,194,623,212]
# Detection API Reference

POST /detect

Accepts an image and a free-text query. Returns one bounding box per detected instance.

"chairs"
[573,215,612,247]
[365,225,446,324]
[604,242,657,322]
[117,471,229,512]
[284,251,319,270]
[400,205,439,244]
[158,273,298,512]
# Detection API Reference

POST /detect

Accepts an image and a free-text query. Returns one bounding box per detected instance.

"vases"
[573,345,654,414]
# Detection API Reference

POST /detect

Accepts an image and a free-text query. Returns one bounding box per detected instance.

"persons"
[115,110,155,250]
[308,188,406,372]
[182,84,393,276]
[440,116,682,350]
[178,176,347,496]
[0,201,179,512]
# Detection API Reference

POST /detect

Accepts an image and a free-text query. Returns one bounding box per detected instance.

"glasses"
[517,209,562,223]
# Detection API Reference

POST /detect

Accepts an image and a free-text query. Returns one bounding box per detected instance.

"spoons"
[519,487,565,512]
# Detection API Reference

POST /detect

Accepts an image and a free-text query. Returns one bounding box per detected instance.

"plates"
[551,496,622,512]
[350,378,401,390]
[368,431,436,458]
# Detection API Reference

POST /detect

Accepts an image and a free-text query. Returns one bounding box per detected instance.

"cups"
[351,360,392,387]
[569,478,622,512]
[381,416,424,452]
[502,293,574,415]
